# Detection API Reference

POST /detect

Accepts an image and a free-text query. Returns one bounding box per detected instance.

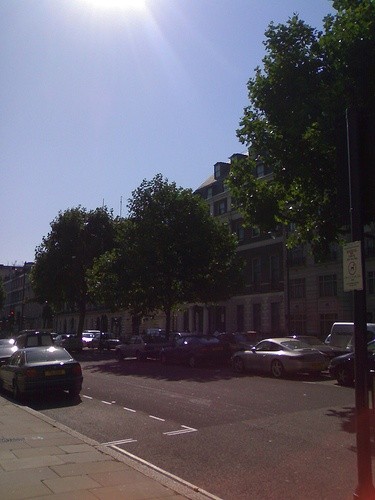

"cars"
[116,322,375,387]
[0,330,121,405]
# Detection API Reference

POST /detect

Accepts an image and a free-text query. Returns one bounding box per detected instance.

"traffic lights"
[11,308,15,316]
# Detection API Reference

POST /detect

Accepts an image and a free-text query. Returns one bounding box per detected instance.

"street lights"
[21,261,34,328]
